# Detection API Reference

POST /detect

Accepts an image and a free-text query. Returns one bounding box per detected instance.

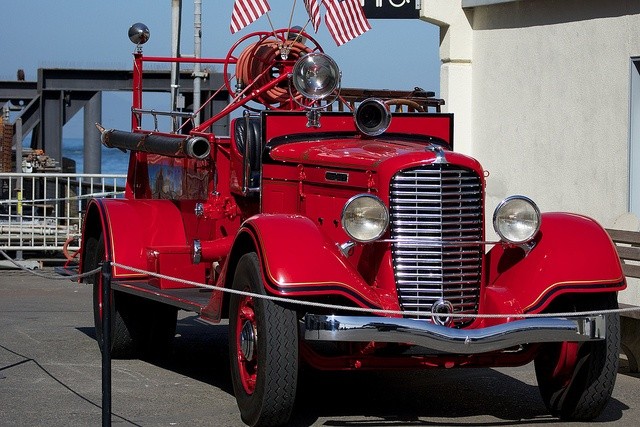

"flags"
[321,0,373,47]
[304,0,321,34]
[230,0,271,34]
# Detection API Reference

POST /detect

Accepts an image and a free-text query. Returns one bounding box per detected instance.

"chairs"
[232,117,261,194]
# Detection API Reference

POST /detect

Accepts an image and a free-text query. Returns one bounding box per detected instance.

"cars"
[60,20,628,425]
